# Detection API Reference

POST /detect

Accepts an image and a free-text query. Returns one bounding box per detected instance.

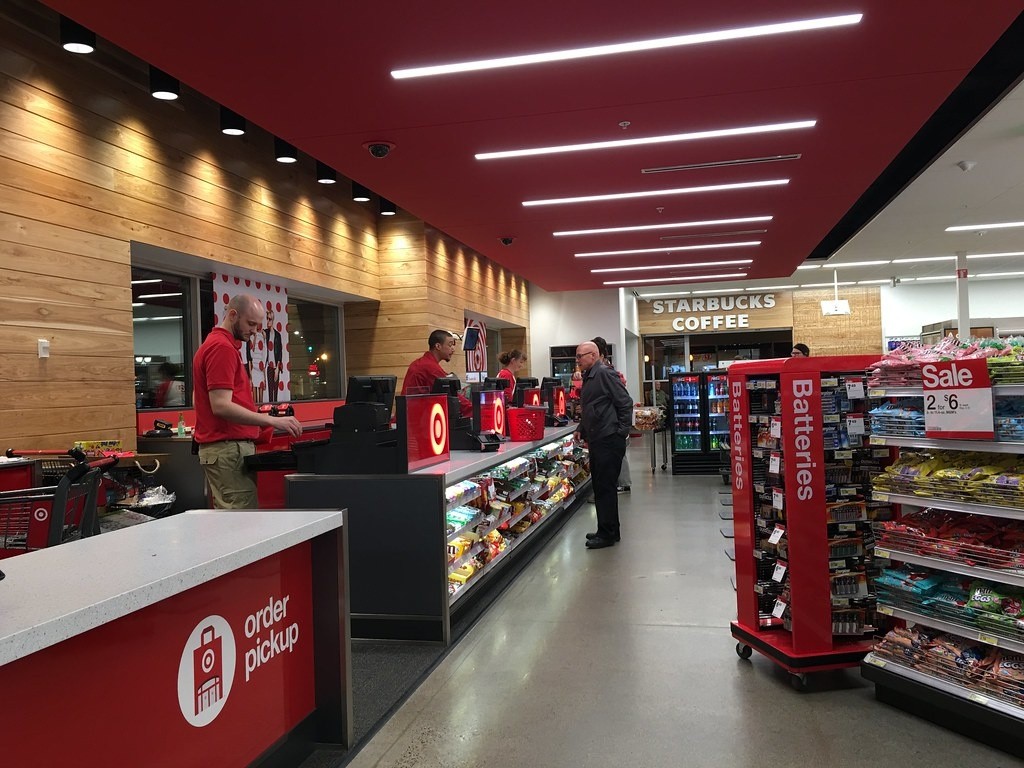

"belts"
[220,438,254,442]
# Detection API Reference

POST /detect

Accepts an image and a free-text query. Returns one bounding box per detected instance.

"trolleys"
[5,447,91,487]
[0,454,120,563]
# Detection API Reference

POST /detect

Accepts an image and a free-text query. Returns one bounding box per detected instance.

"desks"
[138,419,333,514]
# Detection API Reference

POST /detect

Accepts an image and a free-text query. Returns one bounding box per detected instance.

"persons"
[239,308,283,403]
[790,343,809,357]
[573,341,633,548]
[496,348,528,404]
[190,294,303,509]
[576,337,632,493]
[390,330,473,423]
[650,381,671,425]
[153,362,185,406]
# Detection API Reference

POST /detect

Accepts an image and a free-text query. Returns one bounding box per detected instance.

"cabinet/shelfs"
[861,360,1024,757]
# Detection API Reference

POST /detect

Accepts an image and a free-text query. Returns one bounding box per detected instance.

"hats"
[794,344,809,357]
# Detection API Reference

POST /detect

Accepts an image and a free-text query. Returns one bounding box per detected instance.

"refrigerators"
[668,372,729,475]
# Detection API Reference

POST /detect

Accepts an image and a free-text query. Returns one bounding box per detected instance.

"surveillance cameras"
[368,144,390,158]
[500,238,513,246]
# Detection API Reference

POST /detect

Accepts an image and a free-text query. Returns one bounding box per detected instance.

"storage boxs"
[75,440,123,457]
[447,567,475,585]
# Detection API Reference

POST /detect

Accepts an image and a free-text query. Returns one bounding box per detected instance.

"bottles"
[710,435,729,448]
[673,382,699,395]
[710,381,729,413]
[677,436,700,449]
[674,399,701,431]
[709,418,716,431]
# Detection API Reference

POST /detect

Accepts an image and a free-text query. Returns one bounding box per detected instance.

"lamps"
[380,197,396,216]
[274,135,297,163]
[60,14,96,54]
[219,104,245,136]
[352,180,371,202]
[149,64,179,100]
[316,159,337,184]
[958,161,969,171]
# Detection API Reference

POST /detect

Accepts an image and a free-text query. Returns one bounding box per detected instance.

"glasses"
[575,352,592,360]
[791,352,803,356]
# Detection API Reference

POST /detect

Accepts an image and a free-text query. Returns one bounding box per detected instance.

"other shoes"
[586,537,614,548]
[616,486,631,494]
[586,533,620,542]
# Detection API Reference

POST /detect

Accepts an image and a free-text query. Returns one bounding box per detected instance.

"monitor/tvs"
[345,375,399,422]
[512,378,539,407]
[432,378,461,396]
[483,377,511,391]
[462,327,480,351]
[540,377,562,404]
[821,299,850,316]
